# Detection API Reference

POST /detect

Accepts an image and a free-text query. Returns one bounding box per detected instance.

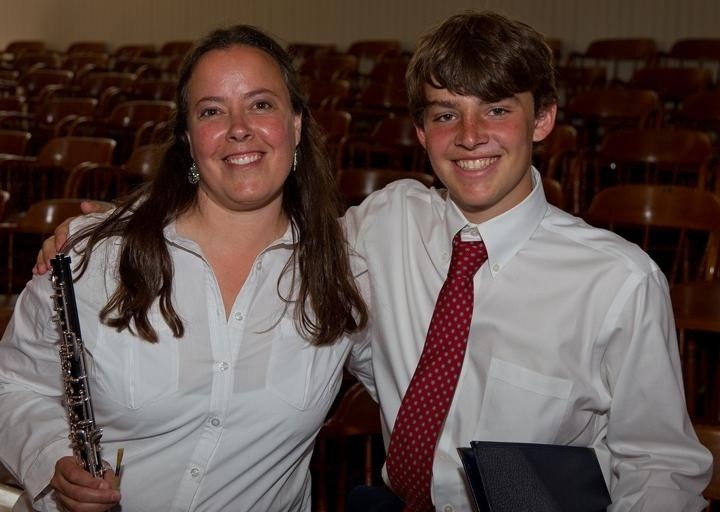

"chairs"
[0,35,720,511]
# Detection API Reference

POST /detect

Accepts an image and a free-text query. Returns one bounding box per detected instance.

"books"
[456,441,612,512]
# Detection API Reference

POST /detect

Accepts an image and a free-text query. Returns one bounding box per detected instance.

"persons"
[0,23,380,512]
[31,7,714,512]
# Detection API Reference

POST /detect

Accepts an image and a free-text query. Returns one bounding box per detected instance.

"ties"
[387,232,486,511]
